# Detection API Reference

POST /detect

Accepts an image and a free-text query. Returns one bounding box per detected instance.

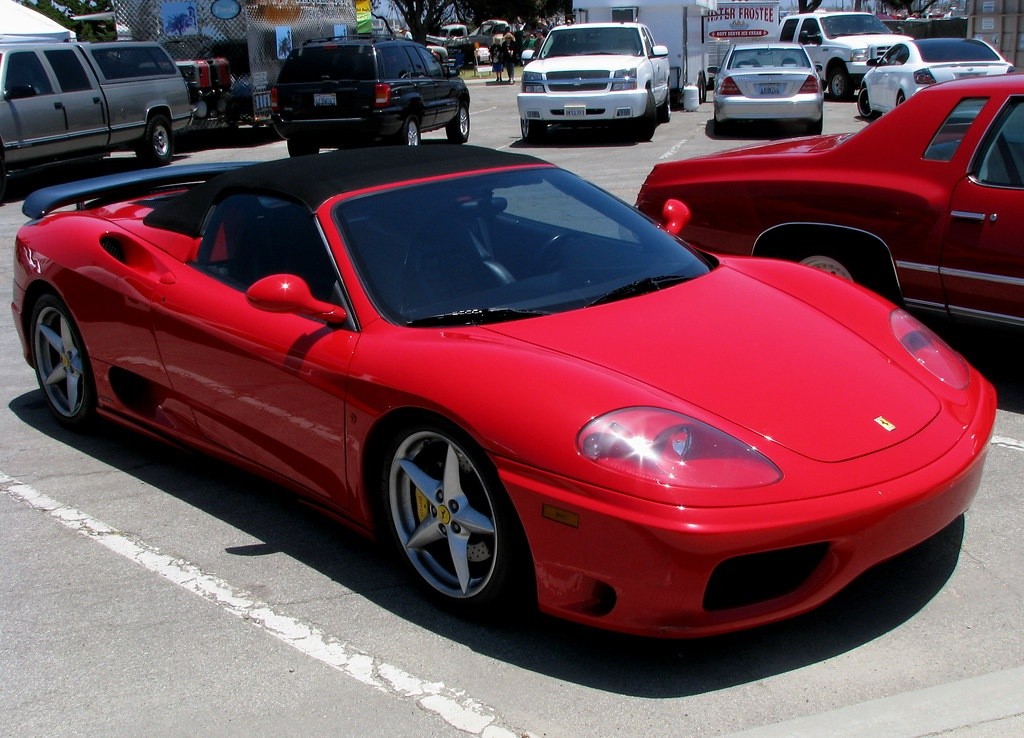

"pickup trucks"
[0,39,200,202]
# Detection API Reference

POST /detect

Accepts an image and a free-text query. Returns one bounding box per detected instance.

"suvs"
[269,33,472,159]
[516,20,671,141]
[776,10,918,102]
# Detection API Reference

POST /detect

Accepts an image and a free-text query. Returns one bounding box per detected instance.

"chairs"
[369,185,501,310]
[736,58,798,67]
[618,38,638,50]
[584,35,602,51]
[220,193,336,304]
[473,48,494,80]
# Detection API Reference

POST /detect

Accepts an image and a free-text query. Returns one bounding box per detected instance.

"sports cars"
[635,71,1024,340]
[8,139,999,637]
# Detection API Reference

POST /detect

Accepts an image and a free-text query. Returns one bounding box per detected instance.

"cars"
[856,37,1018,119]
[707,43,826,135]
[437,20,510,39]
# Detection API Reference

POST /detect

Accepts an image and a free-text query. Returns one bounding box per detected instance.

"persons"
[398,26,412,40]
[489,19,572,85]
